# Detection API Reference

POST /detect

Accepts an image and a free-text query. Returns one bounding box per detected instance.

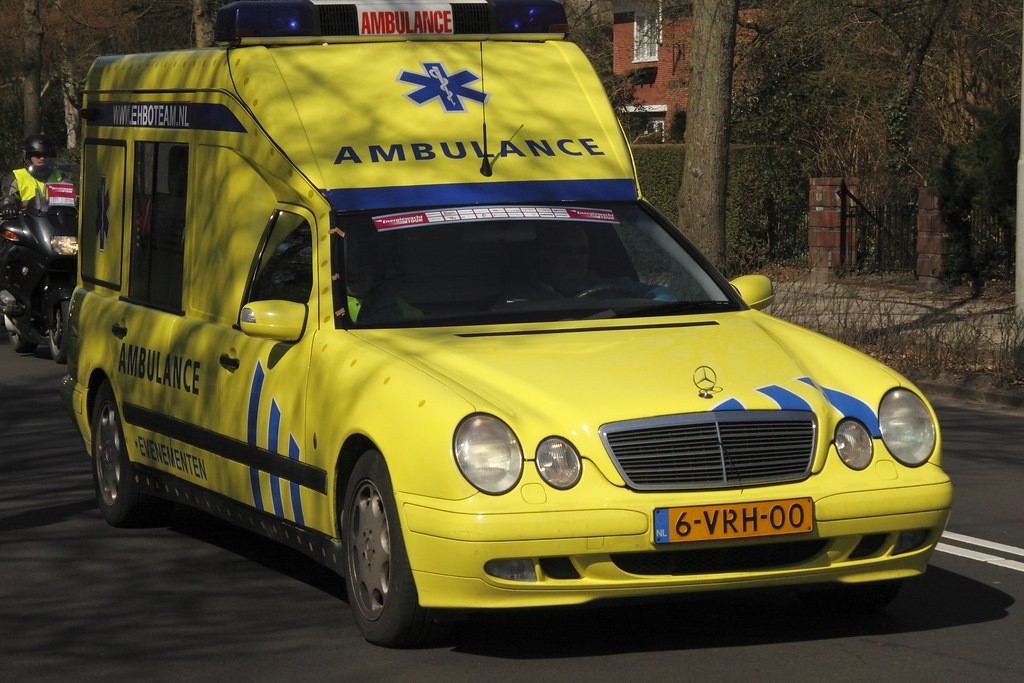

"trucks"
[60,33,953,649]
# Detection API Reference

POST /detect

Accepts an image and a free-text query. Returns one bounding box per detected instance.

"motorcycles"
[0,183,79,364]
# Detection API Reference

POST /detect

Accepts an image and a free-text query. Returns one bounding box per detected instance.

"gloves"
[2,205,20,219]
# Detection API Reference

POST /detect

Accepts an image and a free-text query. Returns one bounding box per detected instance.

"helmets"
[22,135,57,179]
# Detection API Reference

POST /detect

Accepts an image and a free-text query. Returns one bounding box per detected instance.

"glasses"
[549,245,588,257]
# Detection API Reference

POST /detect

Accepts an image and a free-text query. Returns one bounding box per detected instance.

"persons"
[0,133,81,220]
[492,221,676,312]
[341,232,426,324]
